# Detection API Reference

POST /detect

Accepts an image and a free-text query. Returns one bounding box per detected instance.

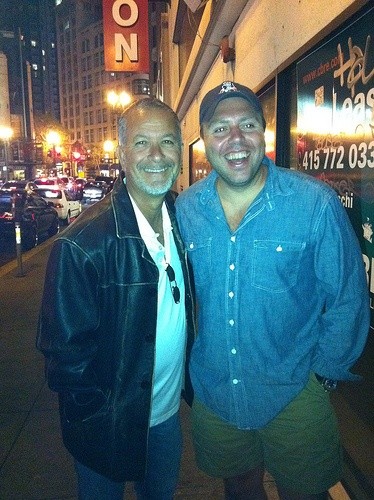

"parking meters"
[11,186,27,278]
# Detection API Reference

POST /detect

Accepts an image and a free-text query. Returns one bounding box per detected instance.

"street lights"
[47,129,61,174]
[70,151,80,177]
[107,89,132,168]
[104,139,115,170]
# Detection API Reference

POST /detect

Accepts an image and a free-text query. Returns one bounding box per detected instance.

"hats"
[199,81,266,125]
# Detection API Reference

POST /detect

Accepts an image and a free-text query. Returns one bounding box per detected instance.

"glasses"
[164,262,180,303]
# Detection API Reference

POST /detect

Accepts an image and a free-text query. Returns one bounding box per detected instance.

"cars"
[0,176,118,250]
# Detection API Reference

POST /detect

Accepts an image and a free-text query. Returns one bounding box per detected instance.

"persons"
[34,98,198,500]
[174,81,369,499]
